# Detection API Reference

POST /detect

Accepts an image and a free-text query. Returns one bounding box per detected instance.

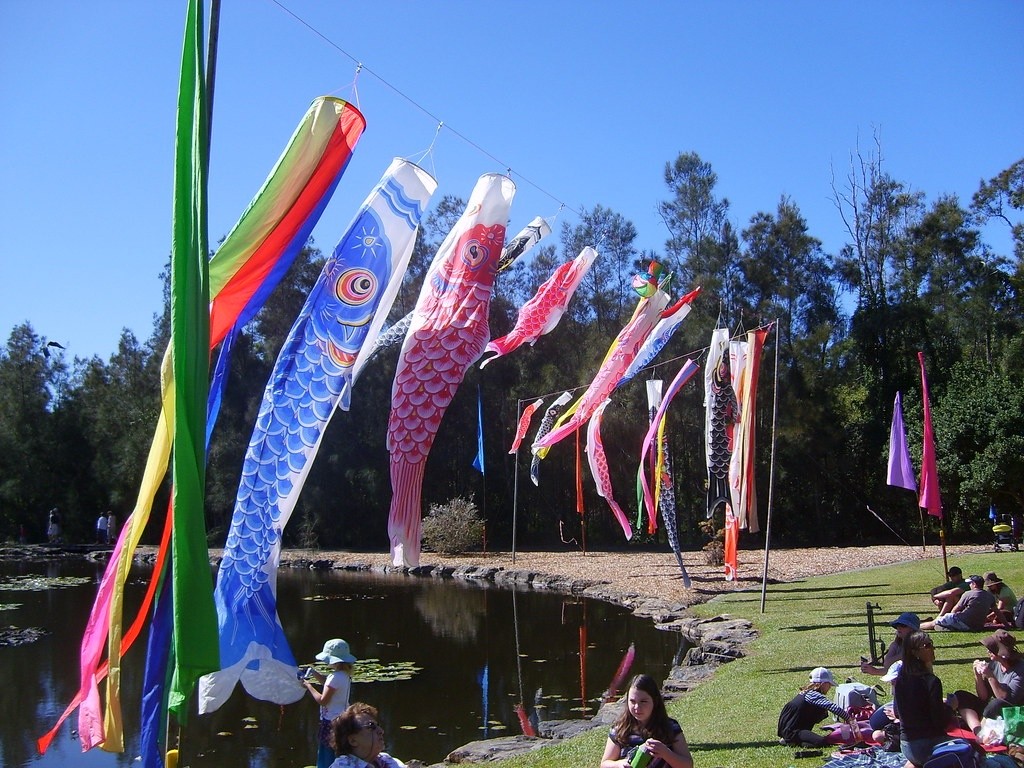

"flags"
[887,391,916,491]
[919,365,944,520]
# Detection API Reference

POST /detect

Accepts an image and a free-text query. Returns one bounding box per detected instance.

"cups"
[839,724,851,739]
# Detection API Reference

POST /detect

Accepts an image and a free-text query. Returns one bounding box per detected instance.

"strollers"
[992,514,1019,553]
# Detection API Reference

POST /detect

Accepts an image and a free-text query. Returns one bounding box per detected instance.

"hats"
[808,667,840,687]
[890,612,921,632]
[314,639,357,665]
[979,629,1021,662]
[965,575,986,586]
[982,571,1003,586]
[946,566,963,578]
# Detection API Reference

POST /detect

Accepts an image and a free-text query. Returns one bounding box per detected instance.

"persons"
[48,508,62,544]
[893,629,958,768]
[777,667,857,749]
[919,566,1018,631]
[861,611,942,731]
[93,511,117,546]
[601,674,693,768]
[329,702,407,768]
[303,639,357,768]
[955,629,1024,736]
[20,524,26,545]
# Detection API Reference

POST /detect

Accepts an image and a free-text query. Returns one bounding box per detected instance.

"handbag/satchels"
[833,677,886,722]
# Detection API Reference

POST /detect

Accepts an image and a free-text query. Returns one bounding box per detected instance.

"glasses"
[359,721,383,730]
[917,642,932,649]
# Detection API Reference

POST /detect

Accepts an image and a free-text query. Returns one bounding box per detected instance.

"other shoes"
[779,738,795,747]
[933,625,951,632]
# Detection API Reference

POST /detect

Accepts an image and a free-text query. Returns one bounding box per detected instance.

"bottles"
[631,743,653,768]
